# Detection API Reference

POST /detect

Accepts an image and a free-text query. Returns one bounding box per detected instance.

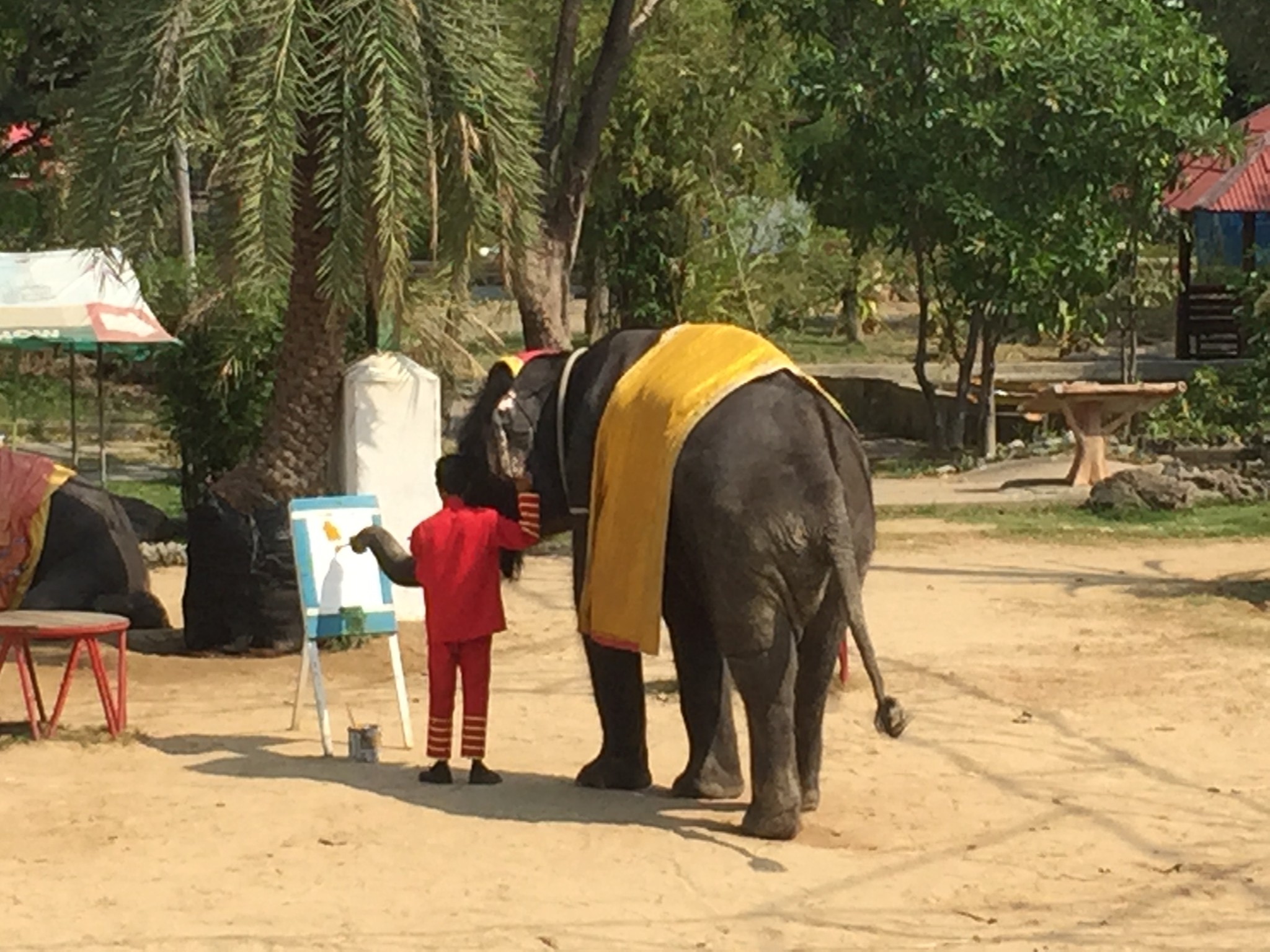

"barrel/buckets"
[347,724,380,763]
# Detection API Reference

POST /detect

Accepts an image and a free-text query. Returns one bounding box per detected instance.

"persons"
[408,453,542,787]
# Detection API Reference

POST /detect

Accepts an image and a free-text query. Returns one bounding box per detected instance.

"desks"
[0,610,131,740]
[1015,382,1186,486]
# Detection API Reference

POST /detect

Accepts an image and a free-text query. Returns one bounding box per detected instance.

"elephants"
[0,446,171,640]
[349,327,909,843]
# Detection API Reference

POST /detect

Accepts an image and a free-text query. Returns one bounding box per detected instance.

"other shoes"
[419,759,453,784]
[467,760,503,785]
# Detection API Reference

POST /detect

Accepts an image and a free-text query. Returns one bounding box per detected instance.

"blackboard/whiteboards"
[287,493,398,641]
